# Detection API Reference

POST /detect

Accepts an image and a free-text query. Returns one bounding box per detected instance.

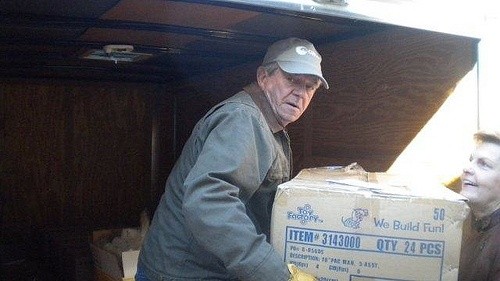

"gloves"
[287,264,318,281]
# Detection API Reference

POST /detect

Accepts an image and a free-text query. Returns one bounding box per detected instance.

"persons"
[134,37,330,281]
[458,129,500,281]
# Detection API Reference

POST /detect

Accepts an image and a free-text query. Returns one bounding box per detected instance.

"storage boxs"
[86,229,147,281]
[270,162,470,281]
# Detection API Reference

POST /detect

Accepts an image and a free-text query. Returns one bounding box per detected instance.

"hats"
[262,37,329,93]
